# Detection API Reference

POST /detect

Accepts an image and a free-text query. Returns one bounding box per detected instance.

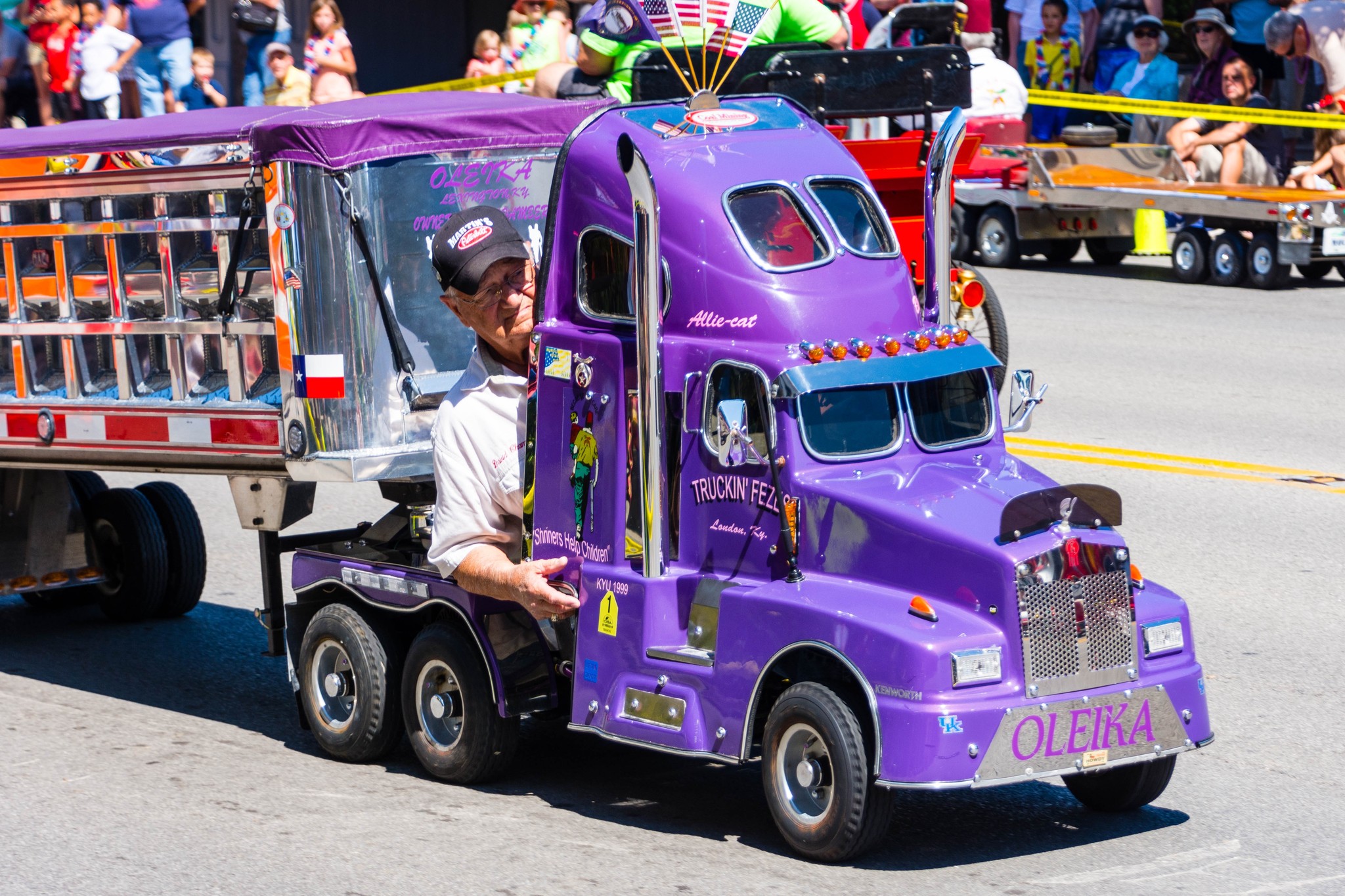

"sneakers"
[1189,218,1227,237]
[1163,209,1186,232]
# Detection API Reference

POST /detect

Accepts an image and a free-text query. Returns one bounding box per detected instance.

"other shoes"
[108,149,154,169]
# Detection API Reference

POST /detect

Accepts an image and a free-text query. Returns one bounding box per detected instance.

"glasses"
[1282,23,1298,57]
[448,251,537,308]
[1133,27,1162,39]
[1194,26,1217,35]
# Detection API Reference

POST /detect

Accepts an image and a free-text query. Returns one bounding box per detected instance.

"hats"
[265,42,291,64]
[432,206,528,296]
[1125,15,1168,54]
[1183,7,1235,40]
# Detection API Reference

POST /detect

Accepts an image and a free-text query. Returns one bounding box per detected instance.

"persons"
[0,0,358,127]
[467,0,1345,226]
[425,205,580,620]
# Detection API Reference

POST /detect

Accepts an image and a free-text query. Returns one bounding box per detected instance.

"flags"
[702,0,771,57]
[283,268,302,291]
[705,0,731,28]
[639,0,676,38]
[675,1,706,28]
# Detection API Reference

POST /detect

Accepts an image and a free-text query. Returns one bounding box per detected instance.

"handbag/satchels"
[231,0,279,35]
